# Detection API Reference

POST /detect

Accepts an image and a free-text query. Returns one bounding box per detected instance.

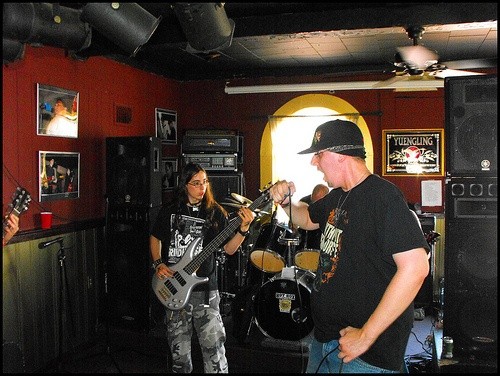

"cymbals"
[220,203,271,215]
[231,193,253,205]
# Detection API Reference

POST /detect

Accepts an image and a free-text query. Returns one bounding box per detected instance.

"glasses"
[187,179,210,187]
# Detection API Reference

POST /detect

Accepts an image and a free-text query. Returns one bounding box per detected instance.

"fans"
[371,24,496,88]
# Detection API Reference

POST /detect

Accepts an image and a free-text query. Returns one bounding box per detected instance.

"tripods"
[76,269,132,373]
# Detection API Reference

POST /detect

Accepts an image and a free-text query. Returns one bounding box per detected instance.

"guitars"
[2,185,32,239]
[152,180,280,310]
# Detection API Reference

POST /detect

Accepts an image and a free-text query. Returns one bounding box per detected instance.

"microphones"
[38,237,64,248]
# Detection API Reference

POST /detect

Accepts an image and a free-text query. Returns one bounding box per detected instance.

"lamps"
[2,3,92,54]
[2,35,24,63]
[79,3,163,65]
[170,2,235,54]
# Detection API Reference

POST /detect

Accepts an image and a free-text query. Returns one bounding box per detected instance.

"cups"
[40,211,52,229]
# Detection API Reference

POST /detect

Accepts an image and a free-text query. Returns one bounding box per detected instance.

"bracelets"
[153,257,164,268]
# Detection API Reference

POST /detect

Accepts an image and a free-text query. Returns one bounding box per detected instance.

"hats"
[298,119,364,155]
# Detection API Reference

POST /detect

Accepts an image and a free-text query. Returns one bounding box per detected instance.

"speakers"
[175,172,247,296]
[108,210,170,333]
[107,135,162,210]
[446,219,498,355]
[444,74,498,221]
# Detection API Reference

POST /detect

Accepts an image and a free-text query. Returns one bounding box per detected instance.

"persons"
[297,186,330,249]
[152,162,257,373]
[2,214,20,247]
[47,100,77,135]
[270,119,430,374]
[46,158,56,183]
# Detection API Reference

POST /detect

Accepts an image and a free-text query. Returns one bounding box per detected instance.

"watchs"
[239,229,250,237]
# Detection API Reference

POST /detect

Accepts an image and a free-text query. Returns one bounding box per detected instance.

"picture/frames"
[382,129,446,177]
[160,157,178,193]
[38,150,80,202]
[37,82,80,139]
[155,108,177,145]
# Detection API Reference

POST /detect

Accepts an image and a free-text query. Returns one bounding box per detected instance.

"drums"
[293,226,321,272]
[249,221,294,275]
[254,265,317,341]
